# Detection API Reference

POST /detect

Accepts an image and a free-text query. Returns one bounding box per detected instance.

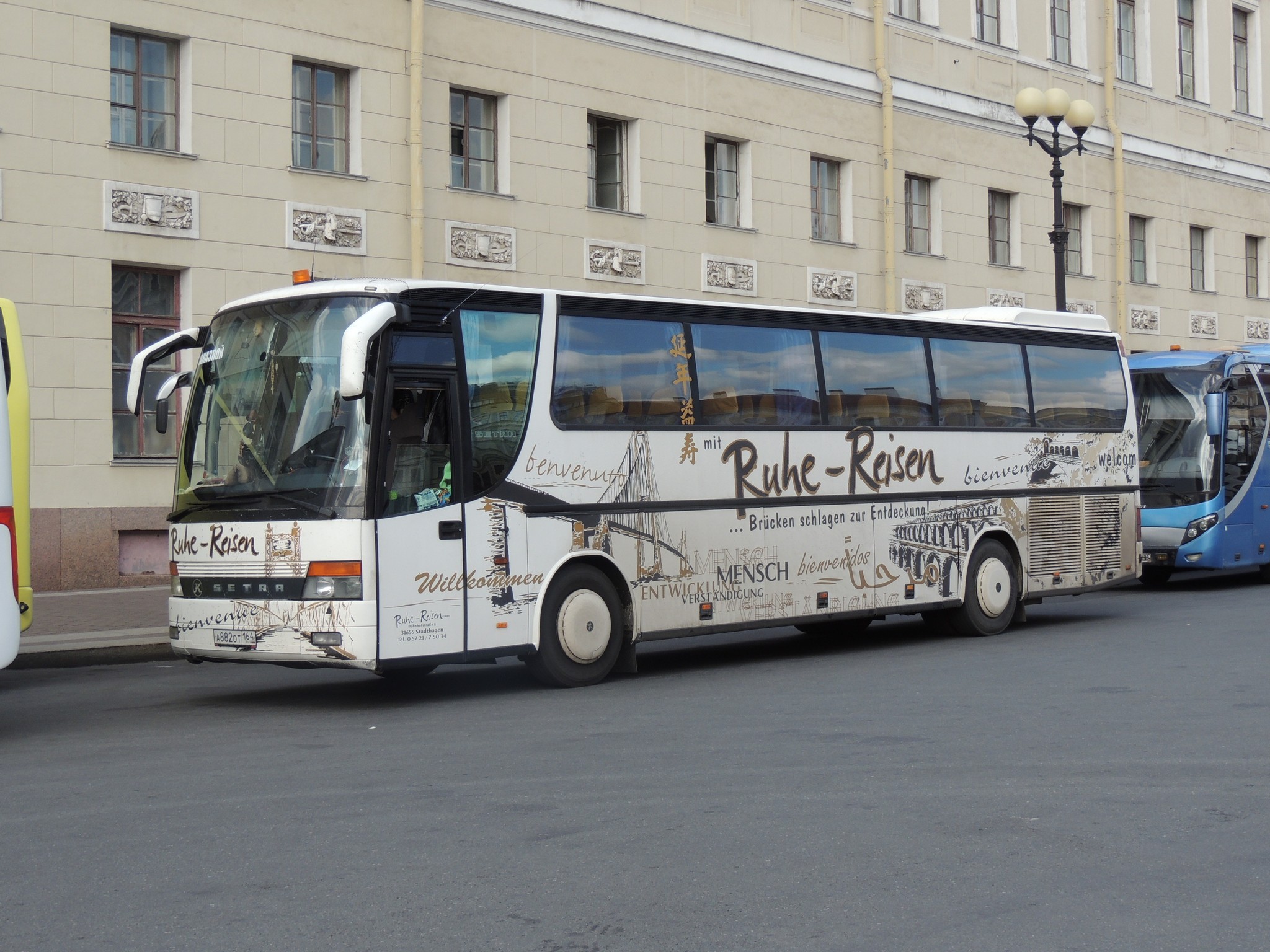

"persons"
[385,390,439,496]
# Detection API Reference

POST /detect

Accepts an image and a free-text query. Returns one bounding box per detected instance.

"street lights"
[1015,86,1097,312]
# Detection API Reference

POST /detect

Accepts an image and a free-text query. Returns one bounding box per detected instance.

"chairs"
[475,380,1101,427]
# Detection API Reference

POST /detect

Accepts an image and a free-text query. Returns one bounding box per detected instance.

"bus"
[0,298,34,670]
[125,268,1145,691]
[1121,343,1270,588]
